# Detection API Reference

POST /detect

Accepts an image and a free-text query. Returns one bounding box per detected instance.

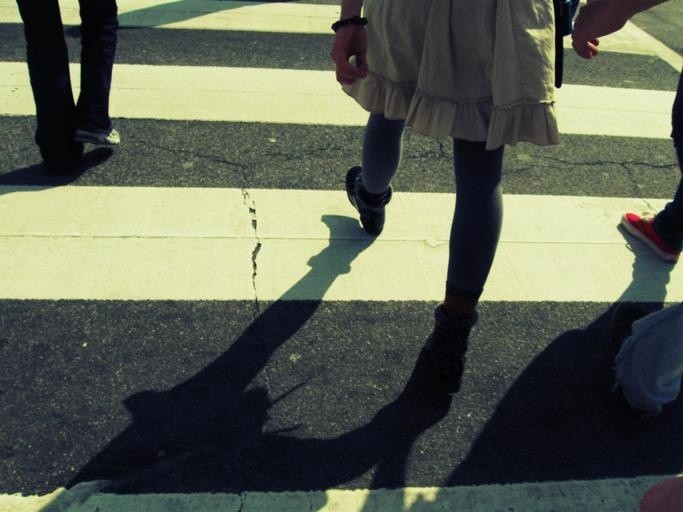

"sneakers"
[77,129,123,145]
[622,212,679,263]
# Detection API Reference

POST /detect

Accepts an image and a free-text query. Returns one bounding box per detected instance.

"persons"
[15,0,124,179]
[571,0,683,264]
[329,0,562,393]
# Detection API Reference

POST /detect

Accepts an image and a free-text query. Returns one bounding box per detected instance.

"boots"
[423,302,475,392]
[344,164,393,234]
